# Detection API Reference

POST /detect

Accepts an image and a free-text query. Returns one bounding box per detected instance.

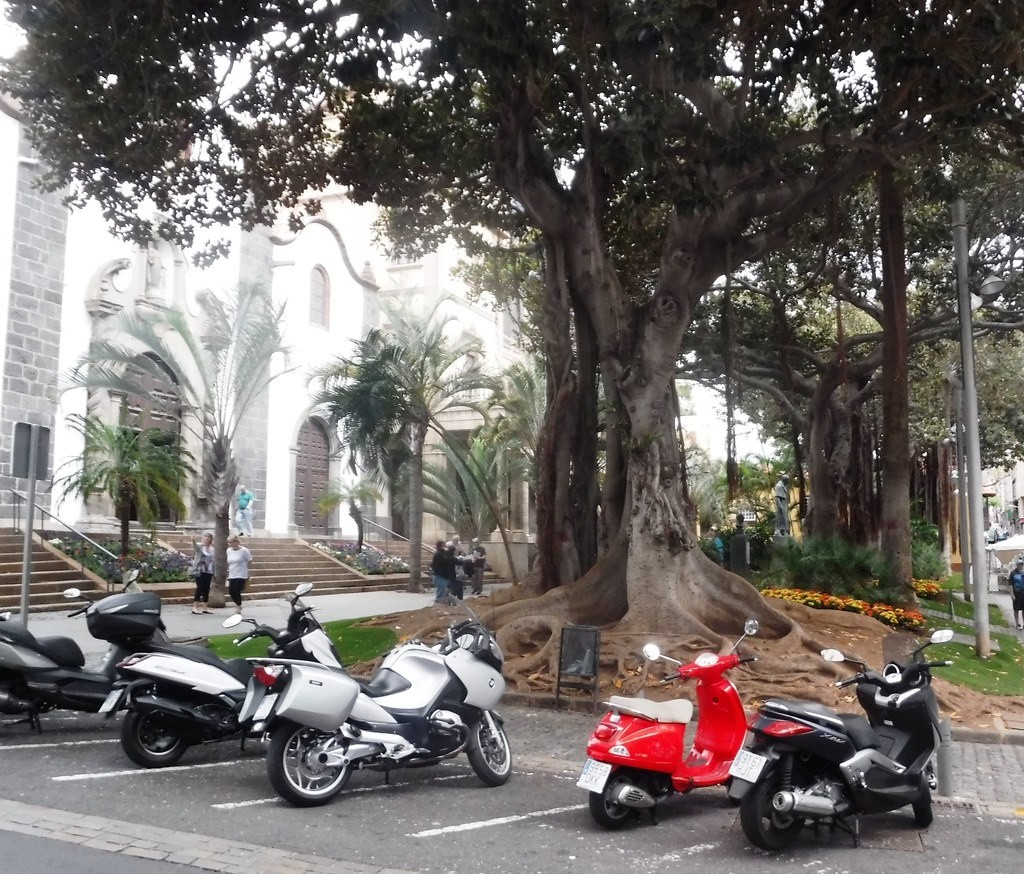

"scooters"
[728,629,957,855]
[0,562,150,735]
[576,617,759,831]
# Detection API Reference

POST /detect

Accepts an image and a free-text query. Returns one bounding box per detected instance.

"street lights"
[950,195,1006,662]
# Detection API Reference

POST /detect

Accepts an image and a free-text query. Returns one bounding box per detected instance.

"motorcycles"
[237,591,513,808]
[89,579,347,768]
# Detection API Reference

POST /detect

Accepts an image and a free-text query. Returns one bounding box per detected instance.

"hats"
[1017,560,1024,564]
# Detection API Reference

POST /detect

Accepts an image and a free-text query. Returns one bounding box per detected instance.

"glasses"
[1018,563,1023,565]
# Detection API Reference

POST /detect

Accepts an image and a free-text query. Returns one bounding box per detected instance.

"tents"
[985,534,1024,574]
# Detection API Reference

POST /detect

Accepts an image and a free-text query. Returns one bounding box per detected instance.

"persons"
[776,475,788,532]
[472,538,486,595]
[191,532,215,614]
[448,546,464,601]
[1009,560,1024,630]
[446,535,461,551]
[225,535,253,615]
[431,540,464,604]
[708,527,724,567]
[736,514,746,535]
[235,485,254,537]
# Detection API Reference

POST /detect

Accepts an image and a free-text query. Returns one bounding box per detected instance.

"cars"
[984,527,1008,544]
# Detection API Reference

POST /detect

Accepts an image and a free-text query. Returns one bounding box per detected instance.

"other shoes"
[476,591,481,595]
[471,589,477,594]
[192,610,203,614]
[203,609,213,614]
[1017,624,1022,630]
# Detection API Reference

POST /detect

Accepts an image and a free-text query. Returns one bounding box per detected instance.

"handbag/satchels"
[187,567,201,576]
